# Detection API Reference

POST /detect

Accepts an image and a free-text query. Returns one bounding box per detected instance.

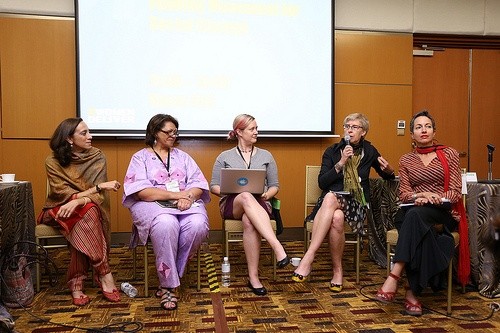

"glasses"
[343,124,363,131]
[159,128,180,138]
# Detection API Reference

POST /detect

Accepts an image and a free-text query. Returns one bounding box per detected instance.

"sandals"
[375,273,402,304]
[154,286,179,311]
[403,286,423,316]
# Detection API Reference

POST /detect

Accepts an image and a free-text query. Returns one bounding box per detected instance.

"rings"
[187,195,189,197]
[432,196,435,198]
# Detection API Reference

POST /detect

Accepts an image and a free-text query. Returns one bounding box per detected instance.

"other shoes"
[94,274,122,302]
[329,276,344,293]
[71,291,90,306]
[291,270,312,283]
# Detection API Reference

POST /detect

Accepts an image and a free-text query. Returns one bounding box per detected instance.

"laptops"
[219,167,266,195]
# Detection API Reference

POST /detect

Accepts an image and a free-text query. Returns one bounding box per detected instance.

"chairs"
[222,196,281,284]
[35,177,96,294]
[132,235,202,298]
[387,167,467,316]
[304,165,361,286]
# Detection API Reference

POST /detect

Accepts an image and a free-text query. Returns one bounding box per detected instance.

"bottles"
[120,281,137,298]
[222,256,231,287]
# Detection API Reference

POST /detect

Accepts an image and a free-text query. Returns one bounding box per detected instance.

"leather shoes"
[247,280,267,295]
[275,246,290,268]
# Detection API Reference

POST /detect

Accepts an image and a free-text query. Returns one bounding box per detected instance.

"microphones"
[344,135,352,159]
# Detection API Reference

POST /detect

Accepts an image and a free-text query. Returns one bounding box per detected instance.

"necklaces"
[240,149,252,153]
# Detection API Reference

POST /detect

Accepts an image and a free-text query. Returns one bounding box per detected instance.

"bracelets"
[413,193,417,201]
[79,197,87,208]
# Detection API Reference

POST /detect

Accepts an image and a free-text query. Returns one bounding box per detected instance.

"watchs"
[390,170,395,176]
[96,184,102,193]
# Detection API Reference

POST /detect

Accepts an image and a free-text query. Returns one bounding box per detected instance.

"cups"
[290,258,301,266]
[0,174,15,182]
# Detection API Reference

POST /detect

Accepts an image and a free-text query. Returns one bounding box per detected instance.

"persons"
[121,114,211,311]
[376,110,462,316]
[37,118,121,306]
[208,114,291,296]
[292,113,395,292]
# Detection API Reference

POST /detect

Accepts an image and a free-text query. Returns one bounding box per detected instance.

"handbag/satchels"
[270,196,284,235]
[0,240,60,309]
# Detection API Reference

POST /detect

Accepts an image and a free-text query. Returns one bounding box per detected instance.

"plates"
[0,181,19,186]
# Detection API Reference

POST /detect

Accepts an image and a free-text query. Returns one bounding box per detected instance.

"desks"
[465,181,500,299]
[0,180,36,299]
